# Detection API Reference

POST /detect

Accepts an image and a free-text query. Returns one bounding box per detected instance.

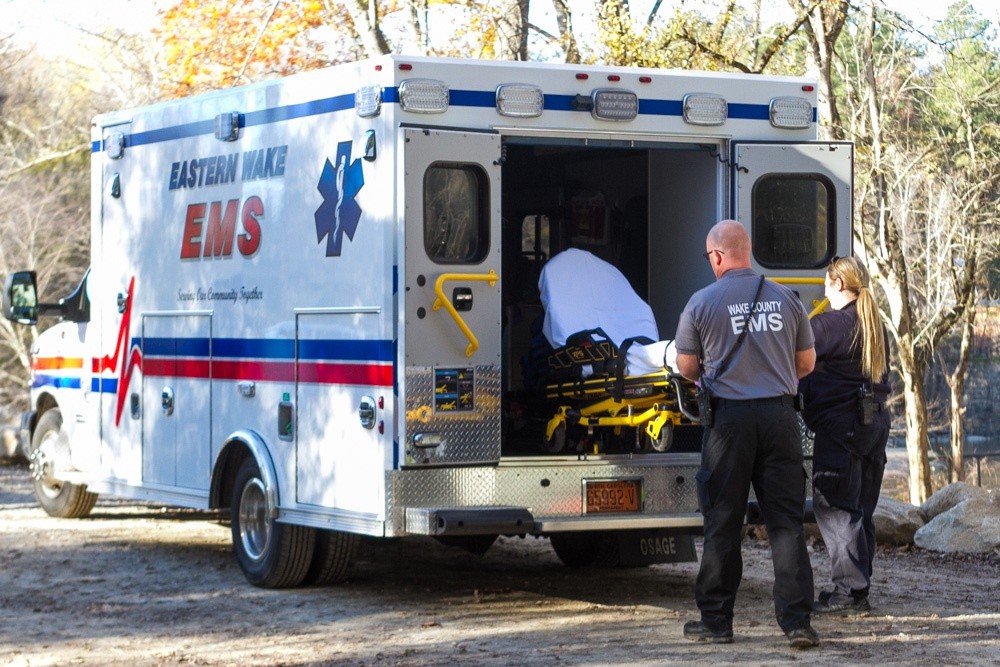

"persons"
[675,219,821,648]
[798,256,891,619]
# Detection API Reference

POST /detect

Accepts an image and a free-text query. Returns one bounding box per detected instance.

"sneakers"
[684,620,733,642]
[813,597,871,614]
[818,591,832,600]
[786,626,820,647]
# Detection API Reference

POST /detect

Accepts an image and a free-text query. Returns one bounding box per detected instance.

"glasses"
[703,250,724,260]
[829,256,843,284]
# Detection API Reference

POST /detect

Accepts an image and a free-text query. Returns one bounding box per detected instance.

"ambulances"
[7,52,855,589]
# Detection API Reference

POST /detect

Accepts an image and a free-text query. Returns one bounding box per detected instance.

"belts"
[873,402,887,411]
[711,395,797,410]
